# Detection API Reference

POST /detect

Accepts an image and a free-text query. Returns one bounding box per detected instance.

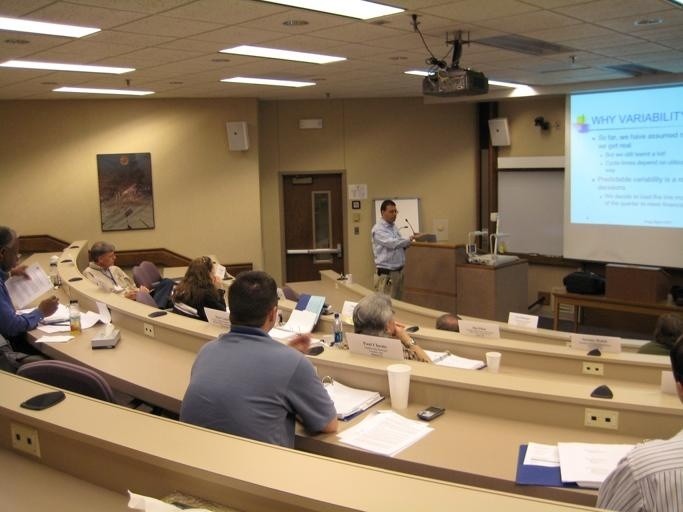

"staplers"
[528,253,538,257]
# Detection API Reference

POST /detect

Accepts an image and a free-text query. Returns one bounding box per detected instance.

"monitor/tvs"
[514,442,637,489]
[284,309,318,333]
[318,375,385,421]
[16,302,71,325]
[295,293,326,325]
[424,347,485,370]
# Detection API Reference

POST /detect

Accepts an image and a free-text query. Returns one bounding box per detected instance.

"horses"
[418,406,445,421]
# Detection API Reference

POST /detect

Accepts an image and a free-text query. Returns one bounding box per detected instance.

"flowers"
[405,218,415,233]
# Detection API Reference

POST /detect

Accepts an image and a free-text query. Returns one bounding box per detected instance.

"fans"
[372,197,420,243]
[496,167,565,259]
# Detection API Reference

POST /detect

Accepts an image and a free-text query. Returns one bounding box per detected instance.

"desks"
[0,233,682,512]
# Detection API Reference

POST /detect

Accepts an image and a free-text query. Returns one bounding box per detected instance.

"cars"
[484,351,501,374]
[385,363,412,411]
[322,335,332,345]
[345,273,352,284]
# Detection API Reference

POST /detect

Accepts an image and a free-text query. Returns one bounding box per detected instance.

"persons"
[638,310,683,356]
[0,225,59,356]
[171,255,227,321]
[369,198,414,301]
[593,334,682,511]
[435,315,462,333]
[179,270,337,450]
[352,292,433,365]
[82,240,151,300]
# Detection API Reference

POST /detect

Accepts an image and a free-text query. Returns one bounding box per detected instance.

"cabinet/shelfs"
[332,313,344,347]
[49,262,61,288]
[67,299,82,333]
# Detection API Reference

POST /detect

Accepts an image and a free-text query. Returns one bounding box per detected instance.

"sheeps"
[489,232,509,258]
[466,229,488,259]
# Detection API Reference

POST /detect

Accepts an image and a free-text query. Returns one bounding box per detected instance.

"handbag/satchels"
[405,339,416,348]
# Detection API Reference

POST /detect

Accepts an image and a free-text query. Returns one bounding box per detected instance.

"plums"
[423,69,488,97]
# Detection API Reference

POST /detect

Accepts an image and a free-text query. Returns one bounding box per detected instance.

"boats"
[225,121,250,152]
[488,118,511,146]
